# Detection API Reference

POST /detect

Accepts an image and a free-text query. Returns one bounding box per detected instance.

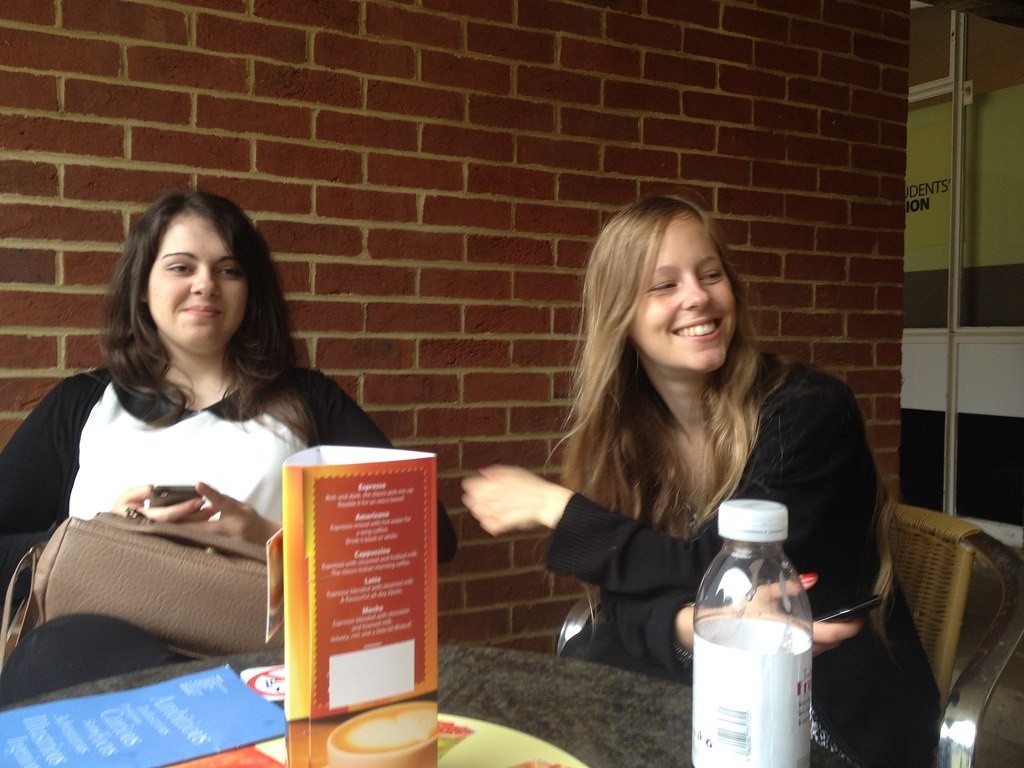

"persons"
[460,193,940,768]
[1,191,456,707]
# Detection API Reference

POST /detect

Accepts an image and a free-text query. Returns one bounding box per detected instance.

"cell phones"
[149,485,202,511]
[812,594,883,623]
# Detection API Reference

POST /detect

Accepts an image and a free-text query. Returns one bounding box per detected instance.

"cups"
[325,701,436,768]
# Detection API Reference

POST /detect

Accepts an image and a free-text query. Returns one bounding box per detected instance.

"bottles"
[693,499,810,767]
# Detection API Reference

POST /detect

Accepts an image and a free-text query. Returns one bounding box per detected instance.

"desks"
[0,641,852,768]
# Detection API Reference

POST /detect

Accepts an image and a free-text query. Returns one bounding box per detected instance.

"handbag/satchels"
[0,509,297,664]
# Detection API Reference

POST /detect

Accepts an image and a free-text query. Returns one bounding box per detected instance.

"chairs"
[556,503,1024,768]
